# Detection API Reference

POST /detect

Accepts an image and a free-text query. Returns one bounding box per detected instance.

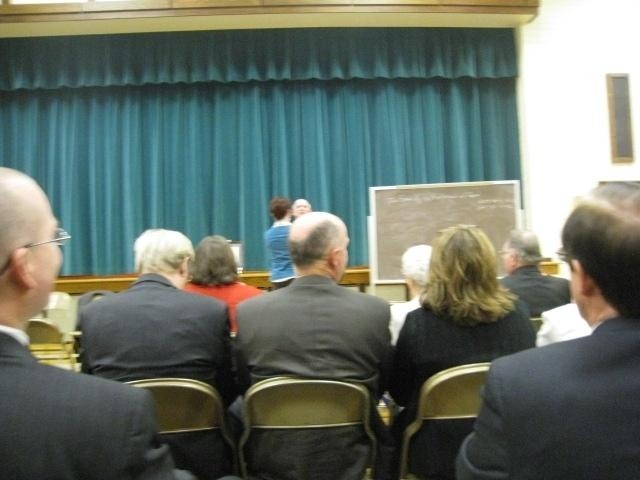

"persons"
[492,227,570,315]
[264,197,293,288]
[79,229,231,480]
[394,225,536,478]
[231,212,391,479]
[1,163,198,480]
[536,247,590,346]
[185,235,264,330]
[390,243,434,341]
[290,199,310,221]
[456,181,639,480]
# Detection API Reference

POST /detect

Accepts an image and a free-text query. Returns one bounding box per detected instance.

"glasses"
[1,227,73,278]
[498,249,511,258]
[556,246,596,268]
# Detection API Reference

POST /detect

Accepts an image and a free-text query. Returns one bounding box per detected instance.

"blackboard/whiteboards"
[369,179,522,285]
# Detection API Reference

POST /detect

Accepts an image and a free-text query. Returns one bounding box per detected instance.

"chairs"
[123,377,238,480]
[237,373,376,479]
[24,318,83,371]
[400,362,490,480]
